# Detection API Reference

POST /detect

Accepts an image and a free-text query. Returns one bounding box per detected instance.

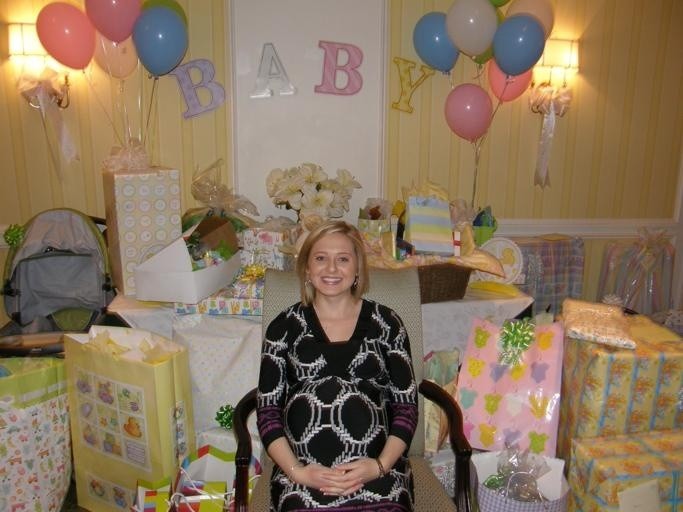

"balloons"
[410,1,558,145]
[36,1,191,81]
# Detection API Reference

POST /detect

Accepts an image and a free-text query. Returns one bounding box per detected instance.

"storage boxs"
[102,165,182,296]
[196,427,261,461]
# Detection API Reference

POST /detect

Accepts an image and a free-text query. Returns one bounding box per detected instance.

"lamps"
[6,23,70,110]
[532,38,580,116]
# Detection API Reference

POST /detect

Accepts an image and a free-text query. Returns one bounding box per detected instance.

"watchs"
[375,457,385,480]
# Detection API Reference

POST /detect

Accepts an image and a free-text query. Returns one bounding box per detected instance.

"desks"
[106,279,533,462]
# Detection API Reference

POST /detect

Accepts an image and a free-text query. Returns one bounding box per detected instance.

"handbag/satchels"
[63,325,195,512]
[454,318,565,457]
[129,476,172,512]
[1,356,74,512]
[356,215,398,259]
[403,194,454,256]
[423,373,459,452]
[172,444,262,512]
[471,453,571,511]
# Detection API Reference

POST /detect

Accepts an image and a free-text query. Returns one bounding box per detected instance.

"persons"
[258,219,419,511]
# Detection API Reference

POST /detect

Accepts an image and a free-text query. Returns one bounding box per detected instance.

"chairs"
[232,267,472,512]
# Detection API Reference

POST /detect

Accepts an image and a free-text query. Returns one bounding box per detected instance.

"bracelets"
[285,459,303,481]
